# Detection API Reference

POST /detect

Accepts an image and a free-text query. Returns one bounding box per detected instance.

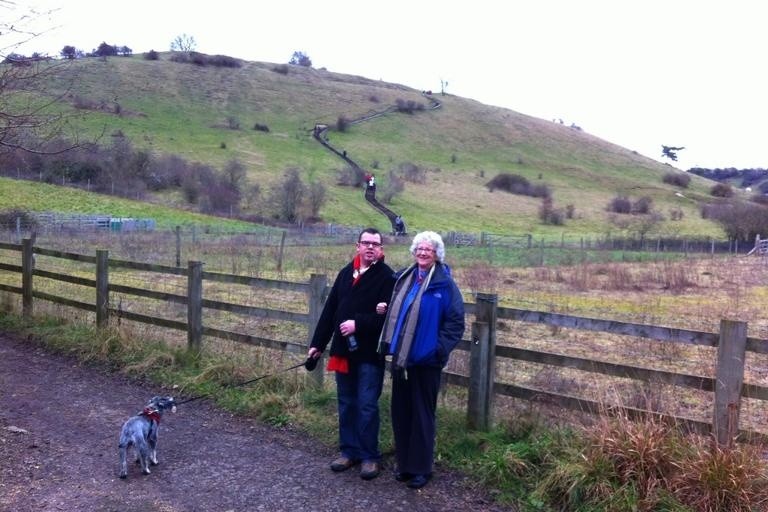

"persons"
[308,229,398,477]
[396,215,403,232]
[376,230,465,488]
[366,173,376,190]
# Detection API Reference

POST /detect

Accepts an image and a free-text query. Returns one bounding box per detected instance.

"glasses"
[417,247,431,252]
[361,241,379,247]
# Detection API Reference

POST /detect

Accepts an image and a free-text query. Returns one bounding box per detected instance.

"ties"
[352,270,362,287]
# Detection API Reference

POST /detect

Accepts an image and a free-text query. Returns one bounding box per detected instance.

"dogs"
[117,393,177,479]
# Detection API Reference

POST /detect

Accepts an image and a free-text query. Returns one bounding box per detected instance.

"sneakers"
[329,447,352,471]
[394,464,432,488]
[359,460,379,479]
